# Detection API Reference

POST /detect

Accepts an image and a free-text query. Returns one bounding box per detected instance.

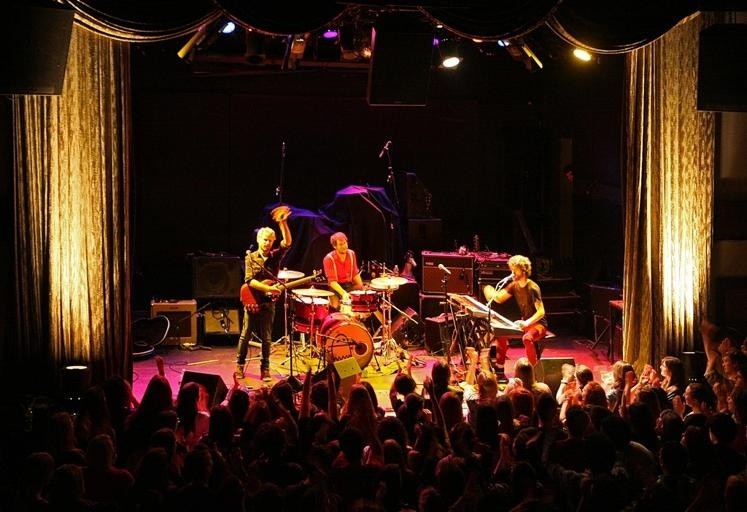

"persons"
[236,210,292,381]
[324,232,388,325]
[3,319,745,511]
[483,254,547,383]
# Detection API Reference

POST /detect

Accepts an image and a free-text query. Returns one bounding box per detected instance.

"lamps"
[175,13,594,70]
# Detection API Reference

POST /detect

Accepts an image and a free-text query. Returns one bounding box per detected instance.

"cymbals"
[277,270,305,279]
[291,289,335,296]
[370,276,408,289]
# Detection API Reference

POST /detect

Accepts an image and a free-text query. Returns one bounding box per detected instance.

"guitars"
[240,269,323,314]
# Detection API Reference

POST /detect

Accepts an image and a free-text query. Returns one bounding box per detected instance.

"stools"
[514,329,557,360]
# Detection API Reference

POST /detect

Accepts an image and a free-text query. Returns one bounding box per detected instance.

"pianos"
[448,292,524,337]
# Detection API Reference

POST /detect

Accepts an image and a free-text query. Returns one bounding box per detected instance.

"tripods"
[180,302,212,351]
[374,291,427,371]
[281,295,327,368]
[256,279,306,365]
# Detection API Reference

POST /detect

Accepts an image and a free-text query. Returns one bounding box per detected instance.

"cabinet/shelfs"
[536,277,582,316]
[419,250,522,356]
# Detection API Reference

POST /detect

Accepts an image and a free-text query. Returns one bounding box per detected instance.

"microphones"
[176,324,180,331]
[352,339,361,349]
[246,250,252,263]
[438,263,452,275]
[282,143,286,159]
[378,141,392,159]
[503,273,515,282]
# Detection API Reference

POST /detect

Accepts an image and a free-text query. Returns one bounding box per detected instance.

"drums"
[339,300,372,319]
[294,297,330,321]
[317,313,374,369]
[350,290,377,312]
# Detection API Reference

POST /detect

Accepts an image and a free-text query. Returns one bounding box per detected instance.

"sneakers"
[261,368,271,381]
[492,363,504,374]
[236,365,244,378]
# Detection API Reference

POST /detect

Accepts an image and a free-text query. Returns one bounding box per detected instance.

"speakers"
[303,357,363,407]
[151,298,197,346]
[177,371,229,408]
[0,3,75,95]
[534,359,575,399]
[365,24,433,107]
[697,23,747,112]
[192,255,241,299]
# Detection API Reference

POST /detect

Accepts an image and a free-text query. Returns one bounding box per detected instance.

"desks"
[608,300,625,366]
